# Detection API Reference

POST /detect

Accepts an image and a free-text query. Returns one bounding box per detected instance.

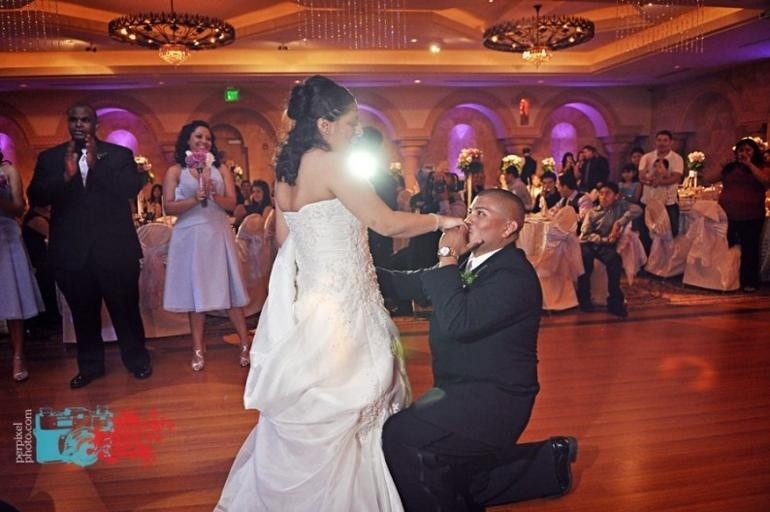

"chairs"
[592,221,633,304]
[137,223,191,338]
[645,201,683,276]
[264,210,275,288]
[60,293,118,343]
[206,213,267,318]
[682,200,741,294]
[527,206,585,317]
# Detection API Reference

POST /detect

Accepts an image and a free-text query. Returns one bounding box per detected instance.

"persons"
[391,130,770,317]
[22,186,60,346]
[215,75,467,510]
[164,119,250,370]
[0,133,47,381]
[375,189,576,510]
[229,178,276,232]
[26,102,152,389]
[150,184,163,217]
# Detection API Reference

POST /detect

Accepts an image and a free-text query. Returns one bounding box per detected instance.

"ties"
[463,258,474,276]
[77,148,90,185]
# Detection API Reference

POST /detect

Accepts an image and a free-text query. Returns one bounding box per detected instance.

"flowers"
[390,162,401,175]
[135,157,154,183]
[688,152,704,170]
[457,148,484,173]
[502,156,524,175]
[733,137,768,152]
[543,159,555,171]
[186,152,215,168]
[226,160,242,181]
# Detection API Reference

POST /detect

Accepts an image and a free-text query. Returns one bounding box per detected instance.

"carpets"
[0,277,770,361]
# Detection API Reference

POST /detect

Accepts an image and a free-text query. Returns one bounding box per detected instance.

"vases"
[688,170,697,187]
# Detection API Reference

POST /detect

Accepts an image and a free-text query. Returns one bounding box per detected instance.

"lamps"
[482,4,595,70]
[109,0,236,68]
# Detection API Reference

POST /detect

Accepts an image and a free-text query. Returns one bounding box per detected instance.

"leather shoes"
[134,359,151,379]
[550,433,577,498]
[609,307,628,316]
[71,370,106,389]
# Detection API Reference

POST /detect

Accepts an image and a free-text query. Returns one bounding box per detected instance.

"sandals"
[13,356,28,382]
[192,345,205,373]
[238,343,250,368]
